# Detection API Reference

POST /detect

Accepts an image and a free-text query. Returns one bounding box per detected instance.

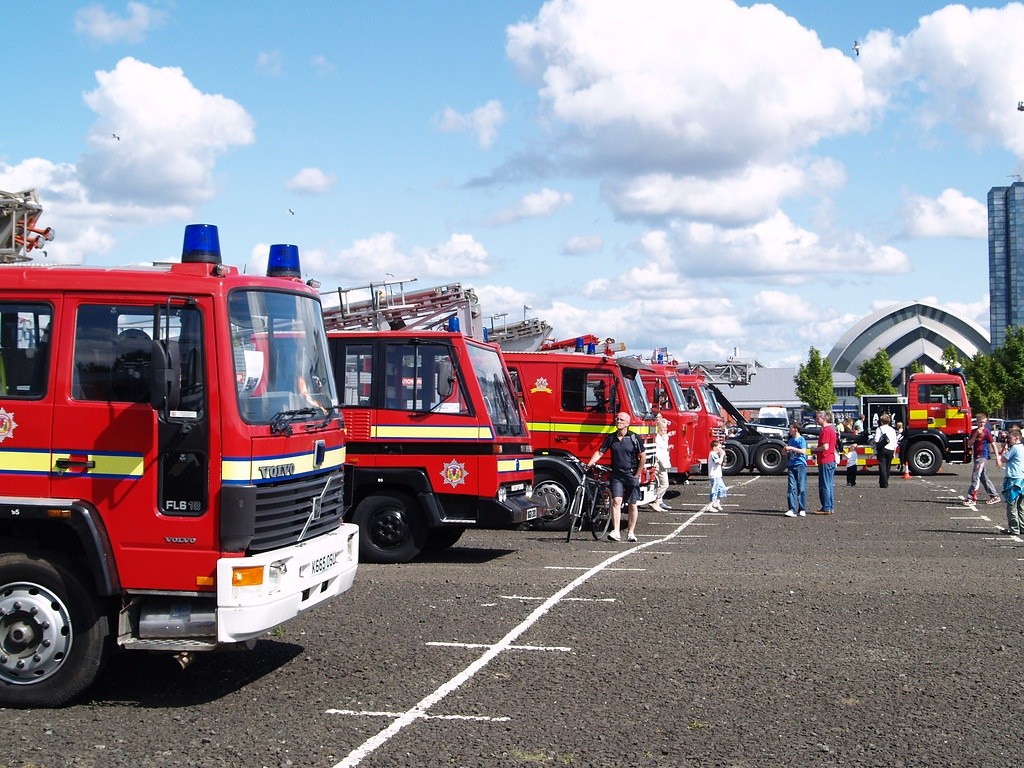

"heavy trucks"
[713,369,974,477]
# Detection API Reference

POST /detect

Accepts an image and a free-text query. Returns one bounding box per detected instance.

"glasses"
[615,417,628,422]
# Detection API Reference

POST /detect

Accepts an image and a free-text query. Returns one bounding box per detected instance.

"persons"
[706,440,726,512]
[854,414,865,433]
[852,425,860,435]
[810,410,837,514]
[648,419,674,512]
[874,414,897,488]
[722,421,735,437]
[785,423,807,517]
[844,444,857,487]
[896,422,904,472]
[963,413,1002,505]
[835,418,853,432]
[999,425,1024,535]
[586,412,646,542]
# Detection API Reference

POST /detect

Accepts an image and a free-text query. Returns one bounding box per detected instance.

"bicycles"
[561,453,613,543]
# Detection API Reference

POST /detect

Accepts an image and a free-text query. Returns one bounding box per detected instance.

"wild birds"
[112,133,120,141]
[852,41,860,56]
[288,208,294,216]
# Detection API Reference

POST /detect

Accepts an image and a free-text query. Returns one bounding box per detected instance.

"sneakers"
[606,531,621,541]
[628,532,637,542]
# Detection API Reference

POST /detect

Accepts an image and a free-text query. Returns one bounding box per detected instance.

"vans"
[758,407,789,427]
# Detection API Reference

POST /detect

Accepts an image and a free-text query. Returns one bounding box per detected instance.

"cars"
[971,417,1022,443]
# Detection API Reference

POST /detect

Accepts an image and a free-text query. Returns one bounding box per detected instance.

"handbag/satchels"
[876,427,890,450]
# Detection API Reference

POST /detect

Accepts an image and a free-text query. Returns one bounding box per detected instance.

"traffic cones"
[861,465,869,471]
[901,462,911,479]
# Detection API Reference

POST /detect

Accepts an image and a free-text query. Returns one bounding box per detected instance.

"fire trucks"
[111,276,543,565]
[344,304,765,525]
[0,190,361,709]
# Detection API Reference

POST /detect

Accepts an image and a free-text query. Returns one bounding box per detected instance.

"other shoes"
[649,502,667,512]
[661,502,672,510]
[985,497,1001,504]
[785,509,797,517]
[800,511,806,517]
[1001,529,1015,534]
[705,506,718,513]
[812,508,834,515]
[962,499,976,506]
[713,503,723,512]
